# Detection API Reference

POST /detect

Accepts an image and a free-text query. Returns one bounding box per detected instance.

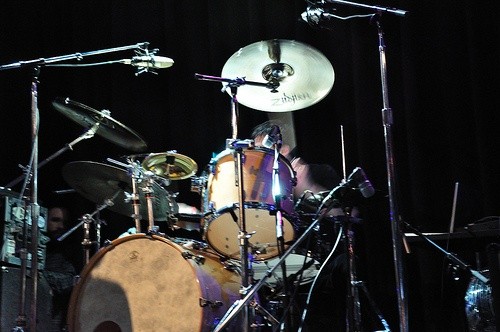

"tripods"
[195,71,281,332]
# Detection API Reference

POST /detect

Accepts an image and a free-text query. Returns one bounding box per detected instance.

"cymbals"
[221,40,335,112]
[60,161,179,221]
[52,97,147,152]
[145,153,198,181]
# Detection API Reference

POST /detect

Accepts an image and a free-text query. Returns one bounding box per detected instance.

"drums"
[67,234,242,332]
[202,147,296,263]
[253,199,323,285]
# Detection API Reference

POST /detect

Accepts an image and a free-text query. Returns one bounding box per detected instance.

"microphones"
[293,193,305,212]
[299,9,331,21]
[348,168,375,199]
[262,124,279,148]
[120,56,175,69]
[398,228,412,253]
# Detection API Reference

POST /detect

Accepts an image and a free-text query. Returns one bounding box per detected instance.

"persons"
[48,204,81,263]
[251,123,359,217]
